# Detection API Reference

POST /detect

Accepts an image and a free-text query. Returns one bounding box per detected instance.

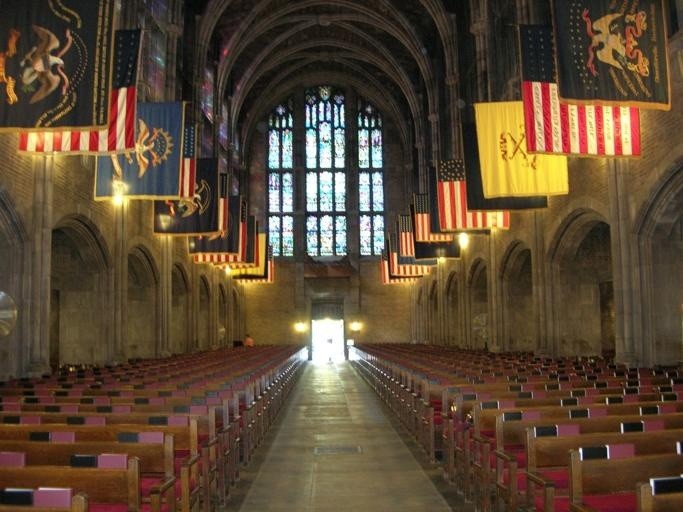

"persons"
[242,334,254,347]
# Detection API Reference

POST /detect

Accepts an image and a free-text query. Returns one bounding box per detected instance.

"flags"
[92,100,185,204]
[456,121,551,213]
[144,118,276,287]
[0,0,120,135]
[513,22,646,160]
[471,96,573,203]
[546,1,672,113]
[10,27,147,154]
[377,159,513,290]
